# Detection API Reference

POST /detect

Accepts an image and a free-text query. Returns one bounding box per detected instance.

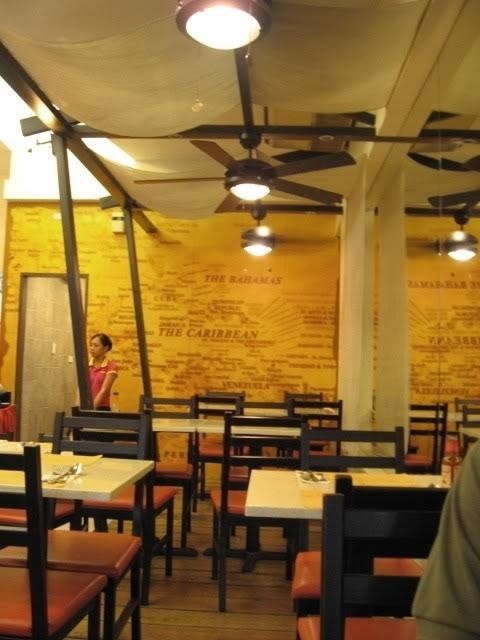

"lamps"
[446,242,479,262]
[172,0,274,50]
[242,235,275,257]
[221,170,272,201]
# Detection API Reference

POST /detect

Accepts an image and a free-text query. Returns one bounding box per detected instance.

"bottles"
[440,438,463,487]
[110,392,121,412]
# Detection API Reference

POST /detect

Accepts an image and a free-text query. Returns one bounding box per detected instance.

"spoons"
[307,467,319,482]
[46,462,82,484]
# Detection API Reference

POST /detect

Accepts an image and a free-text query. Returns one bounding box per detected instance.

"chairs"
[0,386,479,640]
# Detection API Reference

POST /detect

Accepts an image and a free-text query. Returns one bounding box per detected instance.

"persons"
[76,333,118,411]
[411,437,480,640]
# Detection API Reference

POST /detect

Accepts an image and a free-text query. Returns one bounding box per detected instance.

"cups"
[60,451,74,458]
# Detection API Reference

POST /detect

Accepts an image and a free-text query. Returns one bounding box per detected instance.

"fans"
[234,200,324,245]
[188,124,359,217]
[401,208,478,255]
